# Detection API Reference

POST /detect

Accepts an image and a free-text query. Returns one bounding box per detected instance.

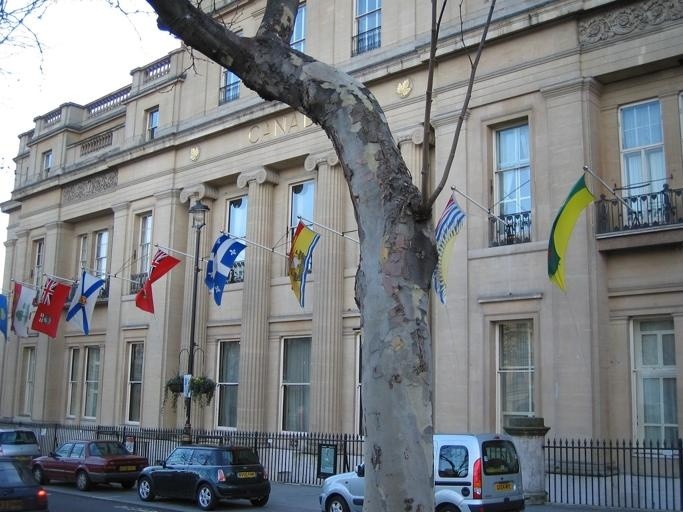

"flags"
[286,221,320,308]
[431,194,465,303]
[134,249,180,315]
[30,279,70,340]
[202,235,247,307]
[10,282,38,339]
[66,271,105,336]
[545,170,596,294]
[0,293,8,341]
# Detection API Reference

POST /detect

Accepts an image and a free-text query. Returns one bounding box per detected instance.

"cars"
[134,443,271,510]
[28,438,149,491]
[0,456,50,512]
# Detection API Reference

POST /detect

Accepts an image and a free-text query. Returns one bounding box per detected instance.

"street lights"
[179,198,210,447]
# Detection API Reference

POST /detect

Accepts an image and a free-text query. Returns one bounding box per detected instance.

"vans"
[0,428,42,473]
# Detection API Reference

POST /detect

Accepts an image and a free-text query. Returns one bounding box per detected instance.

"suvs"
[316,431,528,511]
